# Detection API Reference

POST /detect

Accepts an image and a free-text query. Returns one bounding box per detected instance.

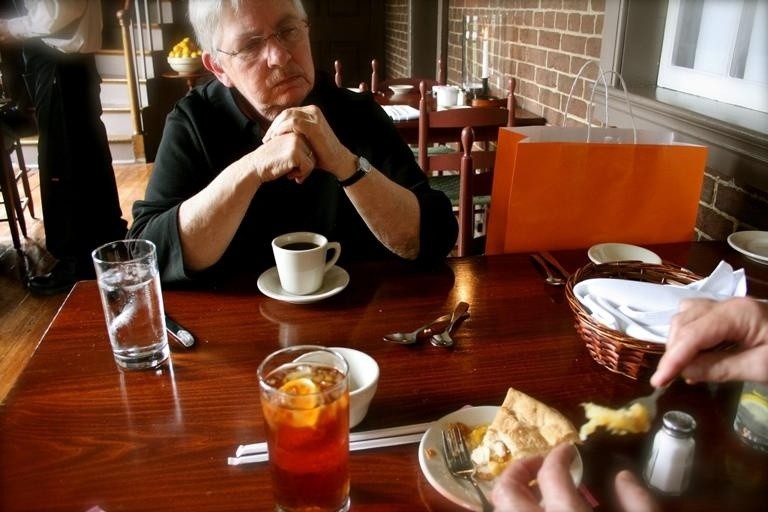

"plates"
[256,265,350,304]
[726,230,768,261]
[388,84,414,94]
[586,242,665,267]
[418,405,584,512]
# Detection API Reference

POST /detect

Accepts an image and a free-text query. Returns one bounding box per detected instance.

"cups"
[90,239,171,369]
[436,84,458,107]
[269,231,342,295]
[255,345,353,512]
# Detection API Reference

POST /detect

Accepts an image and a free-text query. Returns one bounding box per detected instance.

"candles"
[482,28,489,78]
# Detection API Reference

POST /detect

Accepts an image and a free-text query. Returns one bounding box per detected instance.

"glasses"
[216,17,313,60]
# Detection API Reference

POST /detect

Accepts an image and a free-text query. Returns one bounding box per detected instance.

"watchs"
[339,155,372,189]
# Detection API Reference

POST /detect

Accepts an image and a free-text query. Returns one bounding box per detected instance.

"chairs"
[334,59,344,88]
[419,78,516,206]
[371,59,445,96]
[458,127,498,256]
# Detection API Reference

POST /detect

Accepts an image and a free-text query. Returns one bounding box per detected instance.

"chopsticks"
[228,422,437,465]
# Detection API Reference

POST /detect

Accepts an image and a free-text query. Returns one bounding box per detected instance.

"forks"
[440,426,492,512]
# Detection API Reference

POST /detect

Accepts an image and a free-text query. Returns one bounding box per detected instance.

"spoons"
[530,253,566,288]
[380,312,471,345]
[430,302,471,347]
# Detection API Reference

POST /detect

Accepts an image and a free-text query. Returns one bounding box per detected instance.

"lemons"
[168,37,203,59]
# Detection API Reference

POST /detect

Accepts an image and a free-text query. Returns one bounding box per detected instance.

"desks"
[161,70,213,91]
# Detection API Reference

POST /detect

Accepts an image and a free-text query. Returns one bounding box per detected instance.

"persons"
[124,0,459,283]
[0,0,132,297]
[486,291,767,512]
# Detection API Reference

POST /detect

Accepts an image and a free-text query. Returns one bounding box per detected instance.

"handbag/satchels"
[482,60,708,255]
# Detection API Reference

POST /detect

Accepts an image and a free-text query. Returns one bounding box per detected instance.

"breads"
[473,388,577,480]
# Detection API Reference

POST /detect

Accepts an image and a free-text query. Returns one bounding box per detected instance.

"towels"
[572,260,749,347]
[380,104,420,122]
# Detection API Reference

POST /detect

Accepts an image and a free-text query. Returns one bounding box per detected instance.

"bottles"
[457,89,468,106]
[643,410,697,497]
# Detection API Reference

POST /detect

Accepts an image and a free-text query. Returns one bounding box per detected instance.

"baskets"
[562,256,742,386]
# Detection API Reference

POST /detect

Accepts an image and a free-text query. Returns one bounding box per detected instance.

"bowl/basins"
[291,348,380,427]
[167,58,203,74]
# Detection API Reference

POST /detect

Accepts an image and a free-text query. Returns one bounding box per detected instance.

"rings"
[308,144,317,164]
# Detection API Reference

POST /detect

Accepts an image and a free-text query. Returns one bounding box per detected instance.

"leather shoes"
[26,259,91,299]
